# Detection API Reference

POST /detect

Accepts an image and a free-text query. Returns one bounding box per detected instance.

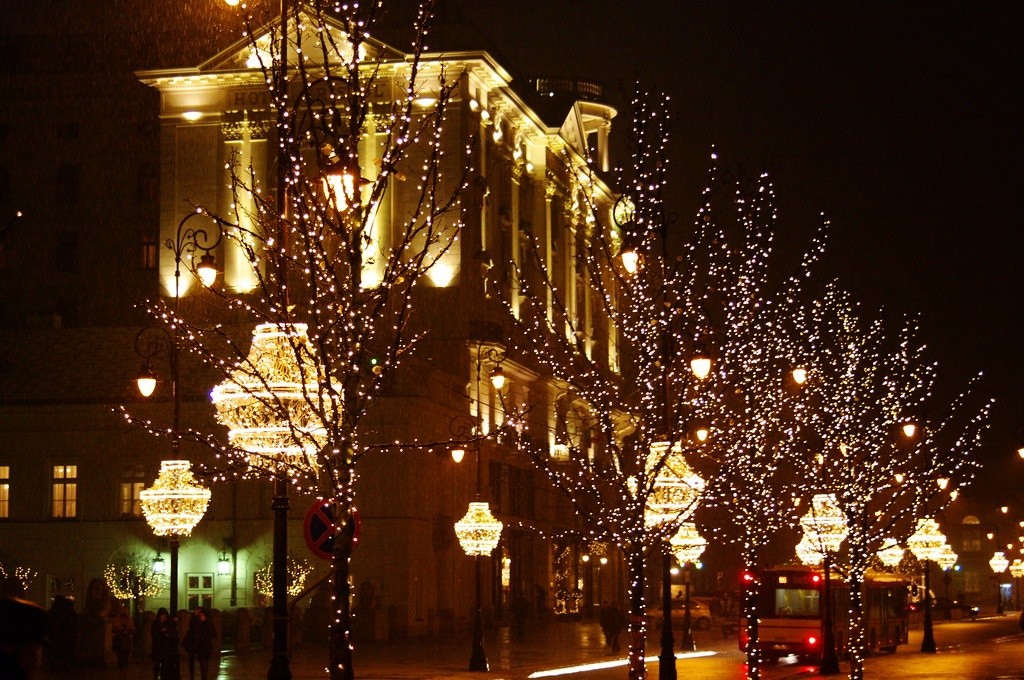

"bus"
[734,560,912,668]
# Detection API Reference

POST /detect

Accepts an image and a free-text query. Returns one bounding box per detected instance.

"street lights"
[449,335,506,673]
[614,187,714,680]
[902,405,947,654]
[986,504,1008,616]
[788,365,851,675]
[263,76,371,680]
[131,210,224,680]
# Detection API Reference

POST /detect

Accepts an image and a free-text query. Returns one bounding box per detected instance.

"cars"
[908,596,978,624]
[629,596,713,631]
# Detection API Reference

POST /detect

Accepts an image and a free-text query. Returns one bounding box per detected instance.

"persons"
[599,590,742,652]
[511,593,534,640]
[0,594,331,680]
